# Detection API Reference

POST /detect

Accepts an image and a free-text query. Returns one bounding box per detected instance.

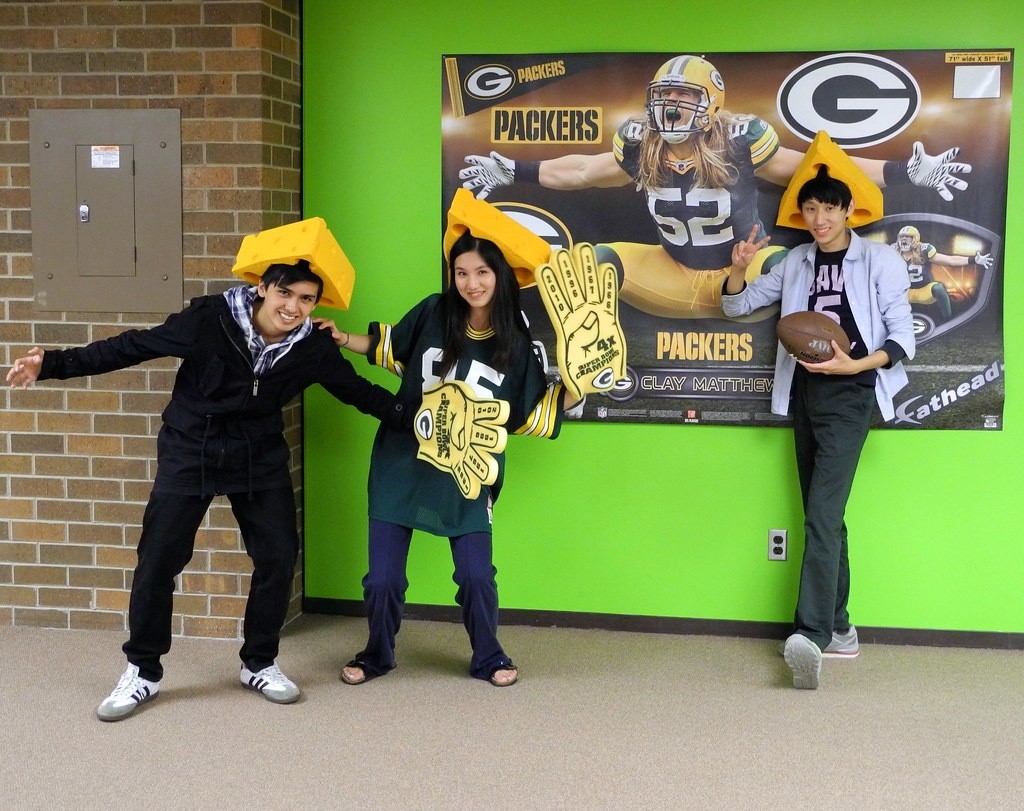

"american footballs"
[776,310,852,365]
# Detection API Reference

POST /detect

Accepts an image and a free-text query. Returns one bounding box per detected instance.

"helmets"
[646,55,726,144]
[897,226,920,252]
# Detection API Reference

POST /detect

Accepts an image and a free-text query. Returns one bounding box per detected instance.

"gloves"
[907,141,972,202]
[459,151,515,200]
[535,243,627,401]
[975,252,993,269]
[413,380,510,500]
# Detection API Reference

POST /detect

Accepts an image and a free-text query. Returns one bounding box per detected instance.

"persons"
[888,225,993,322]
[719,165,914,689]
[6,216,396,723]
[310,237,582,686]
[457,56,973,325]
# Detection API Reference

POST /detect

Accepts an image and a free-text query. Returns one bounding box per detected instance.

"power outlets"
[767,529,787,561]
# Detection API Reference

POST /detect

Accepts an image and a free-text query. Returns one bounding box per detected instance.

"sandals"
[341,659,397,685]
[489,658,519,687]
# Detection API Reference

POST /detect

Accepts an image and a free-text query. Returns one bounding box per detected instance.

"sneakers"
[240,661,301,703]
[97,662,159,721]
[778,624,859,658]
[784,634,822,689]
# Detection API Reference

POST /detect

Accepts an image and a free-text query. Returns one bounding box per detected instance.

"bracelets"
[336,331,350,348]
